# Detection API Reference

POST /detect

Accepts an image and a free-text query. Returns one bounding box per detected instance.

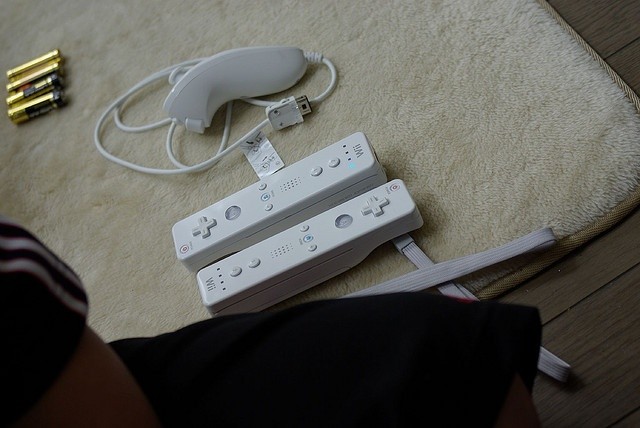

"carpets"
[0,0,640,343]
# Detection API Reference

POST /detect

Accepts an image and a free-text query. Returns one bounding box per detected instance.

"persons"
[0,221,157,428]
